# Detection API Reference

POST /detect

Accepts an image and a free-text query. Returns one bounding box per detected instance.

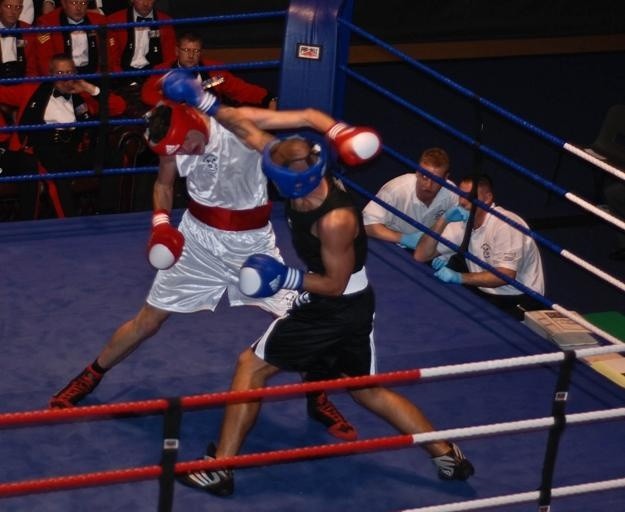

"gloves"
[325,119,383,165]
[147,211,183,269]
[399,208,469,285]
[238,255,302,298]
[161,69,219,115]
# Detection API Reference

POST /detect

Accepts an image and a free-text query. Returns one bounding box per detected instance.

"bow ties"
[137,16,152,22]
[53,90,71,100]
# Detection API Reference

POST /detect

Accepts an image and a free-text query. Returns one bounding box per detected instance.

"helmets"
[261,133,326,197]
[144,100,209,157]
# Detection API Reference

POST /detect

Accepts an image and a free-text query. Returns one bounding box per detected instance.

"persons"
[48,101,381,413]
[176,135,475,497]
[0,0,278,221]
[414,176,548,321]
[360,147,459,257]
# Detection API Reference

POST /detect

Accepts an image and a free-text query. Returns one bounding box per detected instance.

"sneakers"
[174,442,234,495]
[307,393,357,440]
[429,442,474,480]
[48,364,102,410]
